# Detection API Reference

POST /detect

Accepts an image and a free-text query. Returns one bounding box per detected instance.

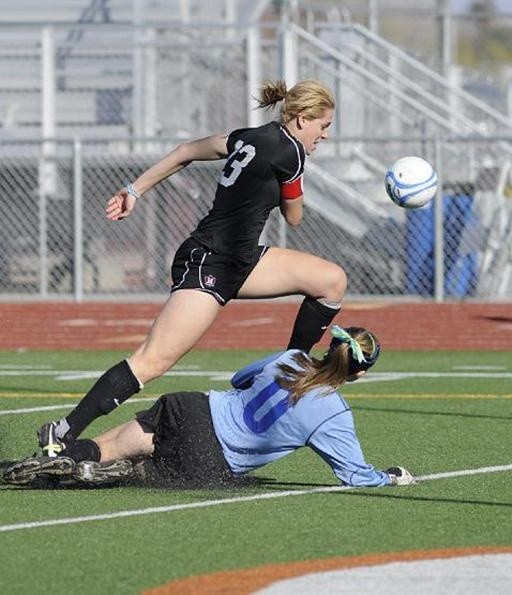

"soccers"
[386,156,439,209]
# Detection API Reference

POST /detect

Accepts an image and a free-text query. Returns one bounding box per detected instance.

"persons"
[1,324,414,489]
[35,76,347,456]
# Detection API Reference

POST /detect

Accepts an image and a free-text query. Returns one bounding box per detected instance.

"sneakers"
[4,422,133,487]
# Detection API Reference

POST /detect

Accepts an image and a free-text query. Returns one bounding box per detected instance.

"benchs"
[0,0,268,156]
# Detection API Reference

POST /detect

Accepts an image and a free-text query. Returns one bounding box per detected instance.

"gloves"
[384,467,419,486]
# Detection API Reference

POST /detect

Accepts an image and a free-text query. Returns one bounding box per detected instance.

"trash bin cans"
[405,185,475,297]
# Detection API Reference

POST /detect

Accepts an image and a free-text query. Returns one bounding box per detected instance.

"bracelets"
[125,184,140,198]
